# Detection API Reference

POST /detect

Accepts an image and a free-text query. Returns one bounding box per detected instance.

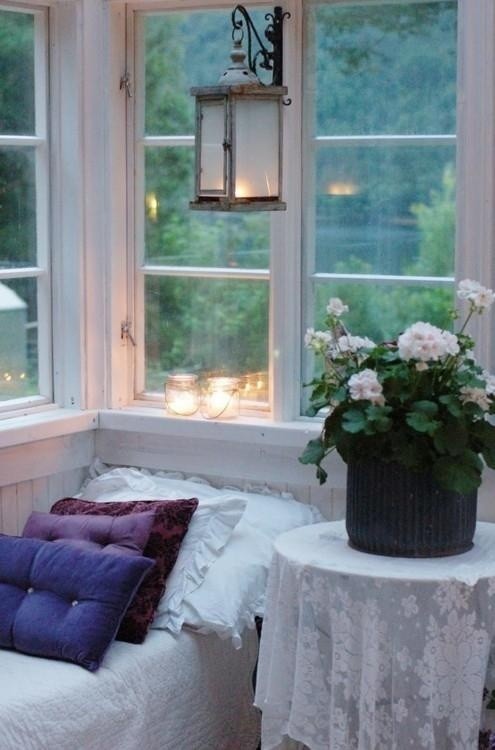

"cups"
[202,375,242,419]
[165,372,200,416]
[242,373,270,410]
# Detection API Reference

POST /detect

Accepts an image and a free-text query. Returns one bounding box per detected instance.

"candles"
[172,393,194,413]
[214,393,234,417]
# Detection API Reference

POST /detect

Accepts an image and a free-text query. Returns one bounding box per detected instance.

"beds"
[1,614,266,750]
[253,520,495,749]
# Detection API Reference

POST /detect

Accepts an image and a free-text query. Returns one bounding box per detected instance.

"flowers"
[298,278,495,497]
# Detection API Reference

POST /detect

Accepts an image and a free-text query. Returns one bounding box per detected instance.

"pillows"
[77,459,324,652]
[19,505,157,558]
[75,466,249,637]
[49,495,201,645]
[1,531,160,671]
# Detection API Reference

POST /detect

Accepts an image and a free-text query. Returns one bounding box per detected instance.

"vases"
[345,457,477,558]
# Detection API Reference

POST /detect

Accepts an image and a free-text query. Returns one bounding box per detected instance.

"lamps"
[189,3,291,213]
